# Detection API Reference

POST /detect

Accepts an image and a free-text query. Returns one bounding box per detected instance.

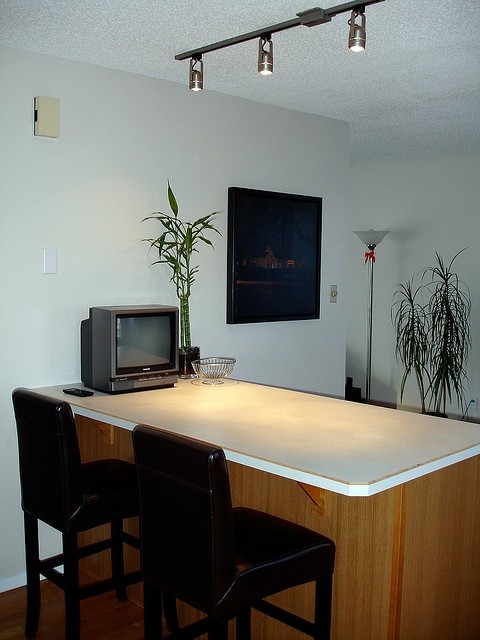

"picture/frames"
[227,187,322,325]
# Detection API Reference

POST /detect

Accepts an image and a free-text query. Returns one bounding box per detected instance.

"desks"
[27,373,480,639]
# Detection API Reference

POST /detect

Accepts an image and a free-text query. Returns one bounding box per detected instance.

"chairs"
[11,385,142,640]
[130,421,336,640]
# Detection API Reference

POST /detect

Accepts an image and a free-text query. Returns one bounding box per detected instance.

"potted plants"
[389,245,472,418]
[137,176,223,380]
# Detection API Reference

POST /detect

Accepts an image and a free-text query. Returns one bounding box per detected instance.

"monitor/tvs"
[80,303,180,395]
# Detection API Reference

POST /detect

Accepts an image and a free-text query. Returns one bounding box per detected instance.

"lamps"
[353,227,390,404]
[347,5,367,53]
[188,52,204,92]
[257,30,274,77]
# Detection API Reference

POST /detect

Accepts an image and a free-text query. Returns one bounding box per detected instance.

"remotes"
[63,388,95,398]
